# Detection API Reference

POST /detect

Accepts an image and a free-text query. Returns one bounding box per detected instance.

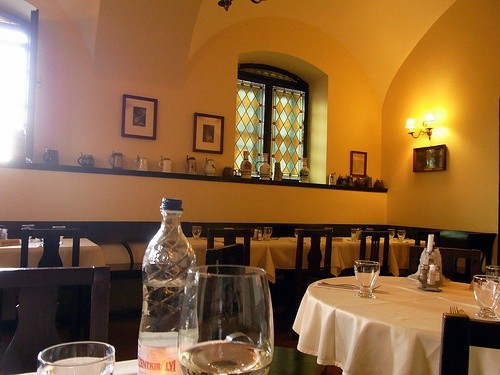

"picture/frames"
[192,111,225,155]
[120,92,158,140]
[350,150,368,177]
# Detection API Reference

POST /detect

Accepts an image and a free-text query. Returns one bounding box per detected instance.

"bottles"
[240,151,310,185]
[136,197,200,375]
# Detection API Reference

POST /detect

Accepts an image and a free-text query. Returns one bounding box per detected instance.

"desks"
[0,236,106,270]
[184,233,416,283]
[290,274,500,375]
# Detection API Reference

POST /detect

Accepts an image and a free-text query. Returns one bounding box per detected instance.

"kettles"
[336,174,384,189]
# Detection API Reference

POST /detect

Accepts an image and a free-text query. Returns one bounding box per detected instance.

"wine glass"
[177,265,274,375]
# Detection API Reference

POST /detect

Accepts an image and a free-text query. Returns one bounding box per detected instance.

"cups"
[350,228,374,241]
[36,341,115,375]
[324,227,333,236]
[191,225,202,240]
[485,265,500,276]
[397,229,406,242]
[294,228,306,244]
[52,226,66,245]
[253,226,273,240]
[387,228,396,240]
[354,259,380,299]
[20,224,35,242]
[473,275,500,320]
[0,229,9,240]
[328,174,336,185]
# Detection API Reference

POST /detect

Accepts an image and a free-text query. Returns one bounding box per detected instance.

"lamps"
[404,112,434,141]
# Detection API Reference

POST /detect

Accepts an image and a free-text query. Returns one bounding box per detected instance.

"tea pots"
[43,149,237,178]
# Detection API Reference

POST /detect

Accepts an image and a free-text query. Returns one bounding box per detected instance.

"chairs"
[293,227,334,279]
[19,227,82,268]
[205,229,251,268]
[203,243,245,275]
[438,311,500,375]
[359,228,393,274]
[0,264,112,375]
[414,229,441,247]
